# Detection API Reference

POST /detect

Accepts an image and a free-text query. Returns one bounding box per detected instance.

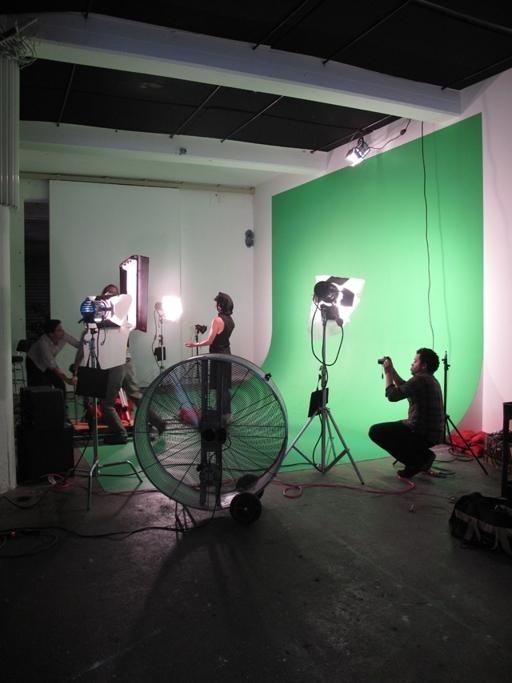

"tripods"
[283,312,367,486]
[390,349,490,476]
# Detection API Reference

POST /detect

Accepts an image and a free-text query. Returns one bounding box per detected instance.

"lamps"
[313,281,340,302]
[345,141,369,167]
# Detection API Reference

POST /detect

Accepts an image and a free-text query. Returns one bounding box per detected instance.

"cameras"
[377,355,391,365]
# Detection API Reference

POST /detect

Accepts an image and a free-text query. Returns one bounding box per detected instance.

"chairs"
[12,338,30,396]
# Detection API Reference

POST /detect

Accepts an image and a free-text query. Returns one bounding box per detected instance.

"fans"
[133,353,286,512]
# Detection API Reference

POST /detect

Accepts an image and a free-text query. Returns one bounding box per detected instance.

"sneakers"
[396,451,440,479]
[103,435,128,445]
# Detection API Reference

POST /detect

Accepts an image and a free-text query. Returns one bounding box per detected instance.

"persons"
[25,316,84,393]
[117,346,169,436]
[184,290,236,424]
[368,348,447,479]
[72,284,129,444]
